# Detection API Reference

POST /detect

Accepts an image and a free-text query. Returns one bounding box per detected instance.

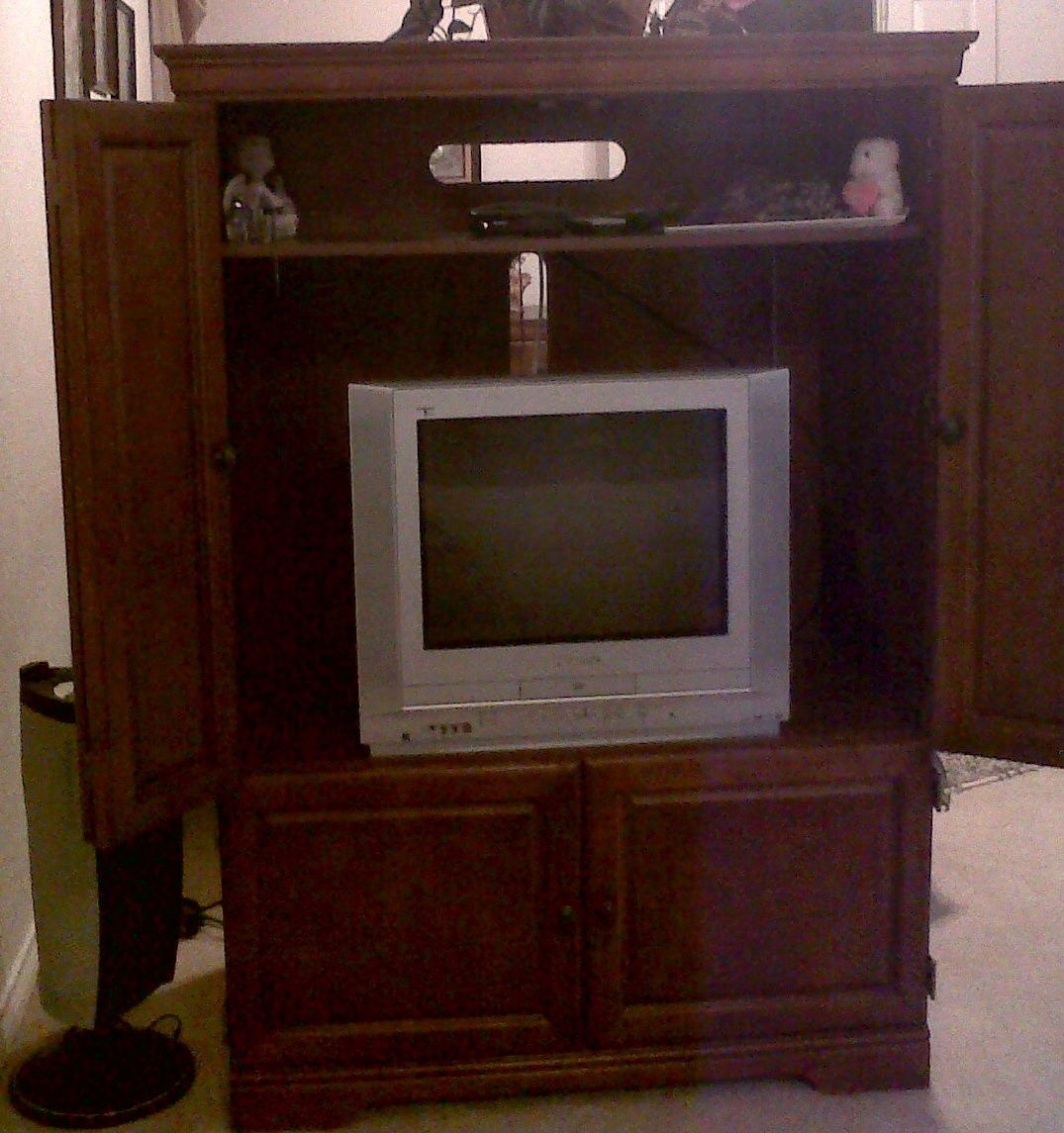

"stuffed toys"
[841,138,905,217]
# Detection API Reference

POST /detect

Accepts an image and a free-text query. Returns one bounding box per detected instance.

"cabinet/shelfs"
[34,71,1064,836]
[221,737,938,1072]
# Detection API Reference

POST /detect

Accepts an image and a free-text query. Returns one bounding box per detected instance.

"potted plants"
[383,0,652,46]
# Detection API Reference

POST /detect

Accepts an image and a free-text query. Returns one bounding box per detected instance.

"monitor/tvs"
[347,367,791,758]
